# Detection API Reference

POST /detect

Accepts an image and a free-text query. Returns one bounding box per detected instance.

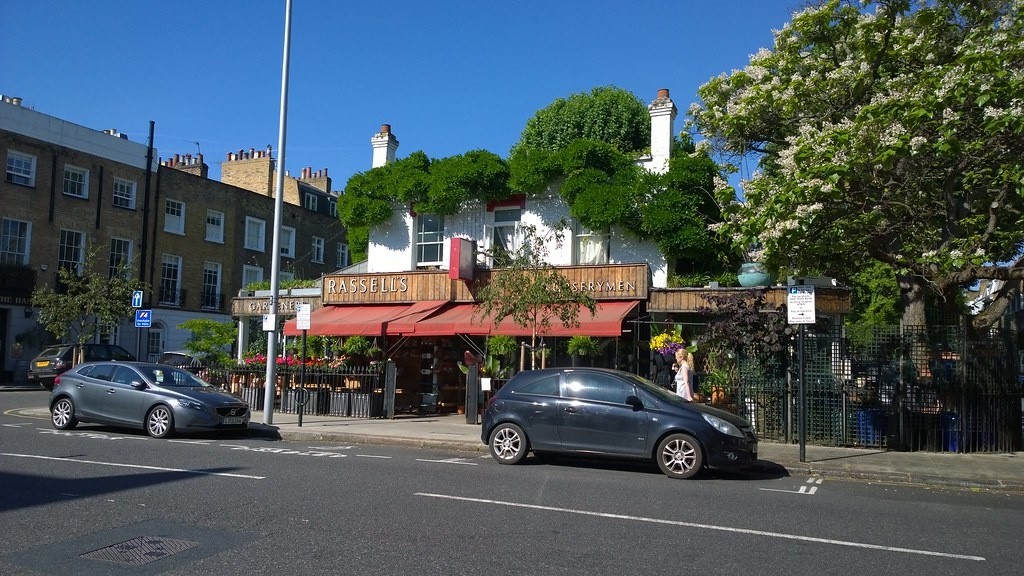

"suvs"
[153,350,211,385]
[27,343,143,392]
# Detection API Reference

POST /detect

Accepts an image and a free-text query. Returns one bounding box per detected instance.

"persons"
[674,348,694,404]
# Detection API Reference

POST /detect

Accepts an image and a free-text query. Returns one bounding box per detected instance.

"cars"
[48,360,251,439]
[480,366,759,480]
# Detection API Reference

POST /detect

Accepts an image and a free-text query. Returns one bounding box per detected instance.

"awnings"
[283,301,640,336]
